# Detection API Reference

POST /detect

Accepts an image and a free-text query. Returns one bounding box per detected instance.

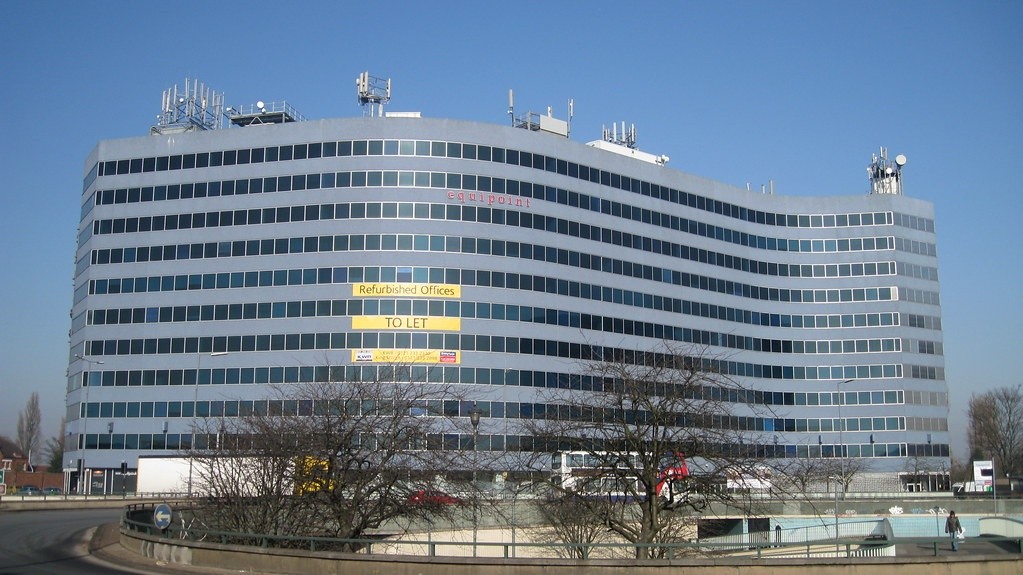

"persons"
[945,511,962,551]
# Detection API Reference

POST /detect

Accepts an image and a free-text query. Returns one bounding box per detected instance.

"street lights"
[185,352,229,500]
[74,353,105,495]
[467,401,483,558]
[836,379,855,504]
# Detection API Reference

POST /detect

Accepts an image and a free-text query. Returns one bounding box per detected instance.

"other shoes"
[952,548,957,551]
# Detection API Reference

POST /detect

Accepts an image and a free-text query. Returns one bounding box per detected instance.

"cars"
[43,487,62,495]
[16,485,41,496]
[404,489,464,508]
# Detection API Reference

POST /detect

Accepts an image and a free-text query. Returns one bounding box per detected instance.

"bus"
[549,451,691,508]
